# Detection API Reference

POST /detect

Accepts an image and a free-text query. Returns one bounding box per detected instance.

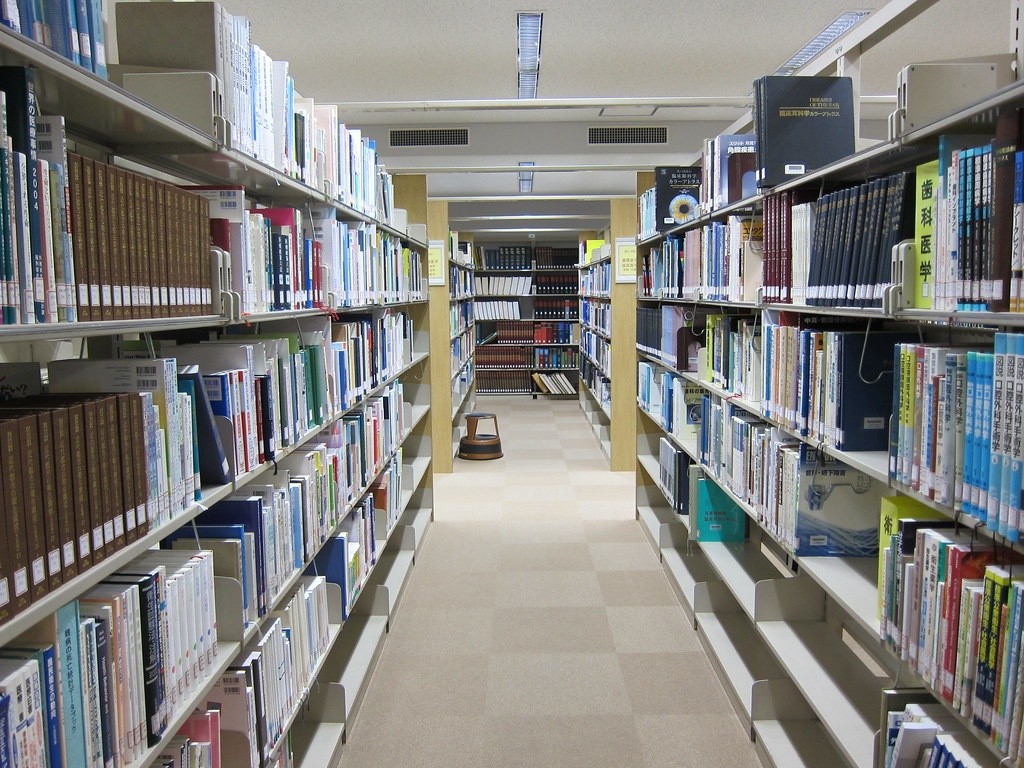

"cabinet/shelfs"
[0,0,1024,768]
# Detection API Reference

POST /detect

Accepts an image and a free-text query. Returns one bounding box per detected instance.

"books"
[474,297,533,320]
[475,276,532,296]
[0,64,215,324]
[177,184,324,315]
[474,244,577,270]
[448,231,474,409]
[475,321,579,346]
[580,354,611,407]
[317,105,394,228]
[580,298,611,336]
[580,327,611,378]
[159,380,403,624]
[476,371,579,394]
[331,308,413,413]
[304,207,422,307]
[0,0,108,81]
[115,3,334,197]
[537,275,579,294]
[0,329,335,628]
[640,75,1023,767]
[476,347,579,369]
[535,297,579,319]
[579,239,611,267]
[581,261,611,296]
[0,550,220,767]
[194,573,330,767]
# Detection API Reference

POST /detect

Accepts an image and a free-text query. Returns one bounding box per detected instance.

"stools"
[458,413,504,460]
